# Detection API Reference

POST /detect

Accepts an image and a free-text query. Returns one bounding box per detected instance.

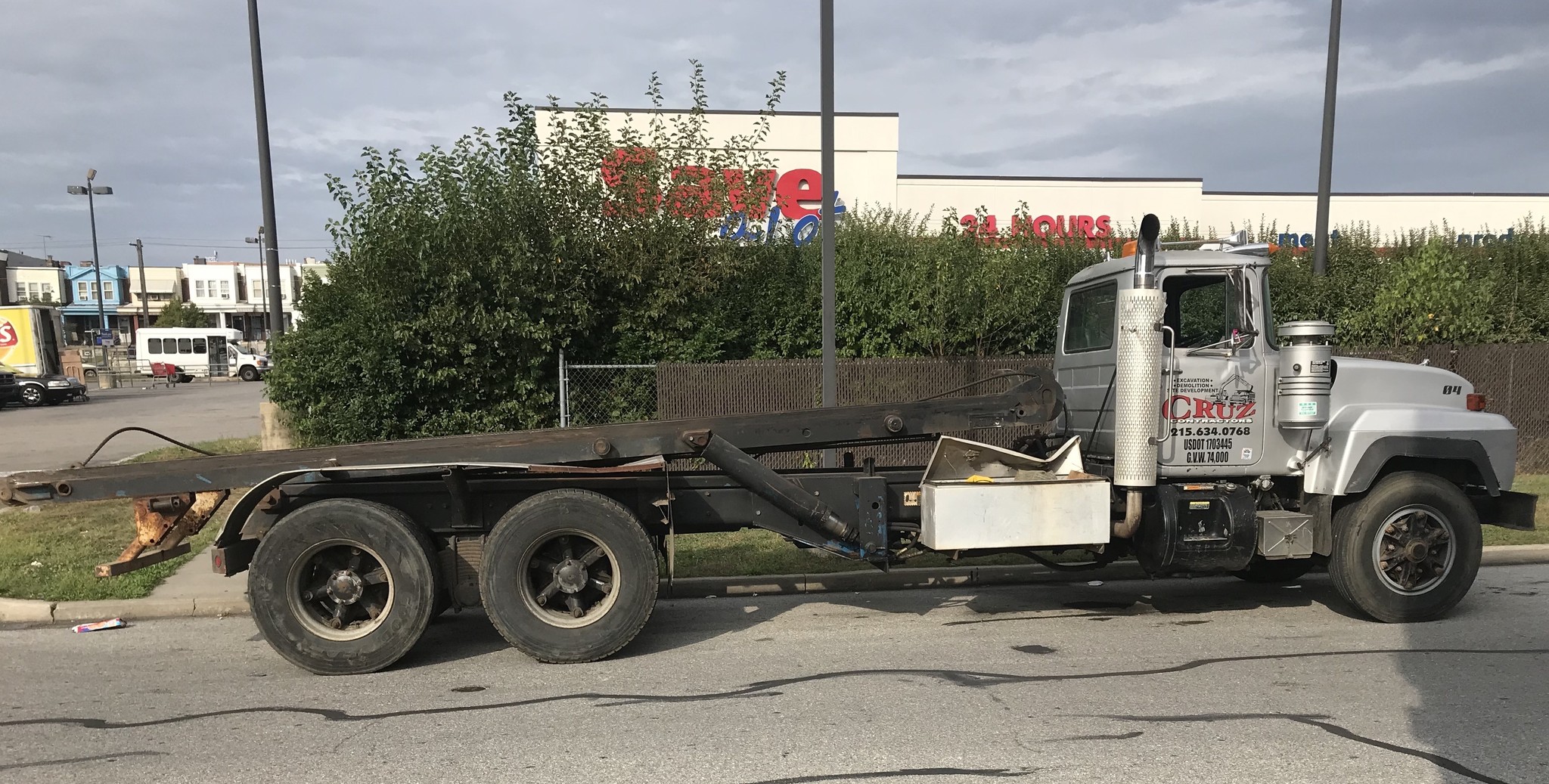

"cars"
[262,354,275,373]
[0,360,86,408]
[81,358,104,377]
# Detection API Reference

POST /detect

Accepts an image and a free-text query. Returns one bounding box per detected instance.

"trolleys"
[149,361,176,388]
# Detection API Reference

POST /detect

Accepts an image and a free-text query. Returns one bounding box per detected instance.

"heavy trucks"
[0,212,1540,678]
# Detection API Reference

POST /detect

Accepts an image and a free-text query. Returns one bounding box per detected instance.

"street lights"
[245,226,270,355]
[67,168,113,371]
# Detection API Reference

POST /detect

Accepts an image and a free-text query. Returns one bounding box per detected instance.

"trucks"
[134,327,271,382]
[77,347,139,379]
[0,302,65,376]
